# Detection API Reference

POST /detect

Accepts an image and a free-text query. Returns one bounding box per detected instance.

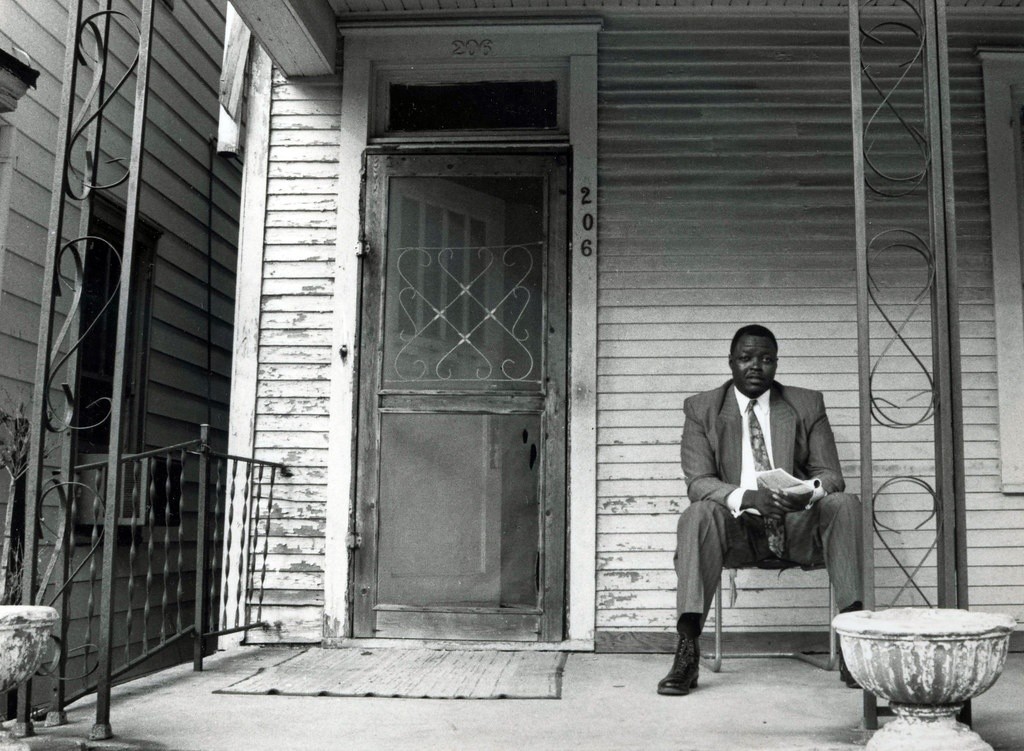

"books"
[755,468,821,499]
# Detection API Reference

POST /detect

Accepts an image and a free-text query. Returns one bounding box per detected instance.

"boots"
[839,650,863,688]
[656,633,700,695]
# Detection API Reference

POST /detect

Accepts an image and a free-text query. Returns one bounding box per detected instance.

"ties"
[747,400,785,558]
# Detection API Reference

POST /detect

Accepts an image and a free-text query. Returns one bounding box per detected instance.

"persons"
[658,324,863,695]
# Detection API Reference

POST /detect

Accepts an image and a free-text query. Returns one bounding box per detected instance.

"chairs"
[698,562,837,671]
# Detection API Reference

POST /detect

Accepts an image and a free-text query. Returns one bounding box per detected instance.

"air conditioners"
[78,451,181,529]
